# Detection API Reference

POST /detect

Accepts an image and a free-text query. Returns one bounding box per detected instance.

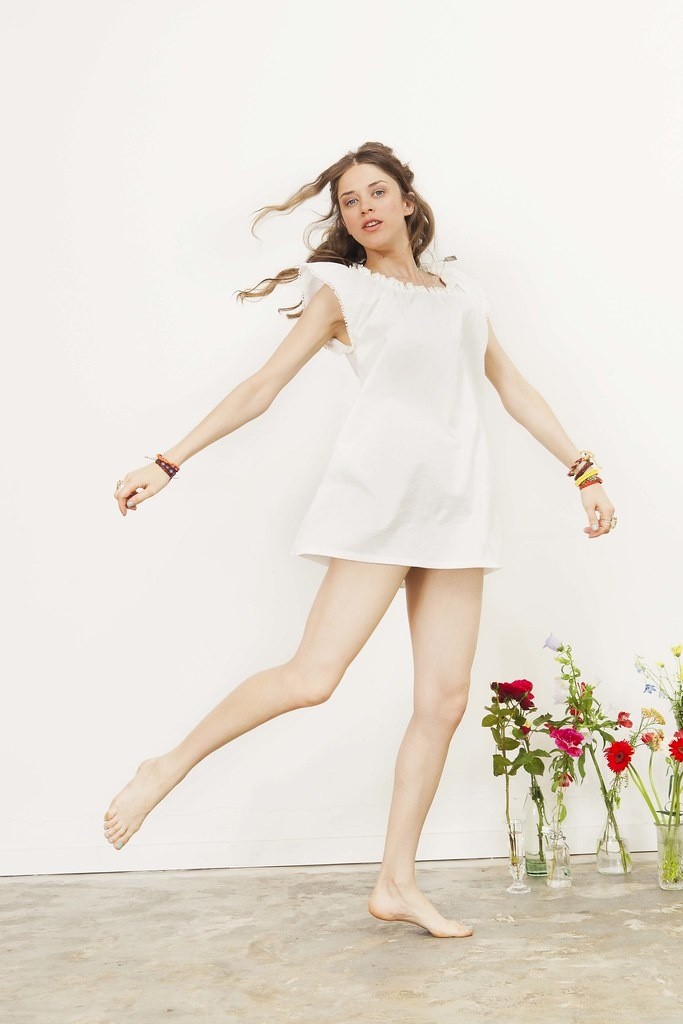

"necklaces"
[419,255,458,289]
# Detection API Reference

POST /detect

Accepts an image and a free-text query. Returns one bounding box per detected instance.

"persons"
[101,142,617,941]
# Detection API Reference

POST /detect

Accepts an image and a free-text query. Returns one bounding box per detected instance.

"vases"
[502,810,681,894]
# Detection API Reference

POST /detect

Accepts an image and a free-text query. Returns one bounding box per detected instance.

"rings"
[600,517,617,529]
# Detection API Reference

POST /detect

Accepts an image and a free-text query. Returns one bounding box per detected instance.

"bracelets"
[155,459,176,478]
[577,476,603,490]
[574,468,599,486]
[573,461,593,482]
[567,450,603,477]
[156,454,180,472]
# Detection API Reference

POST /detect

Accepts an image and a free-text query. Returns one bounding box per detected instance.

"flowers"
[478,633,682,828]
